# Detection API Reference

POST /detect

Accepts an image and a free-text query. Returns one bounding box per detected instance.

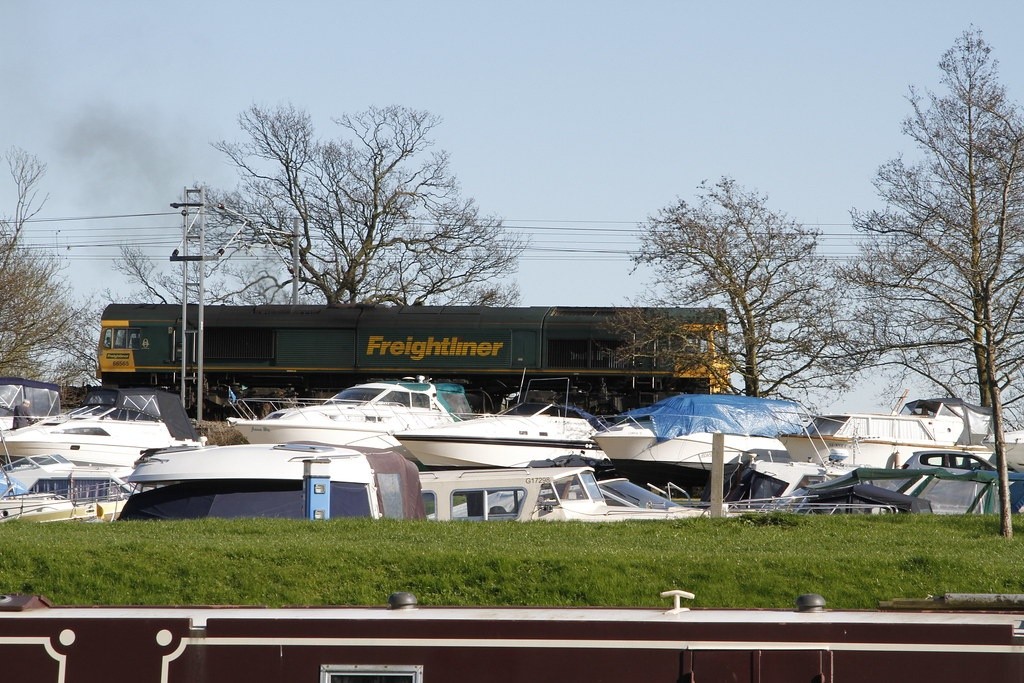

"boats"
[0,371,1024,517]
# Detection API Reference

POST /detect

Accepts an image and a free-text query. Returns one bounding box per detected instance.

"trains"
[95,304,730,421]
